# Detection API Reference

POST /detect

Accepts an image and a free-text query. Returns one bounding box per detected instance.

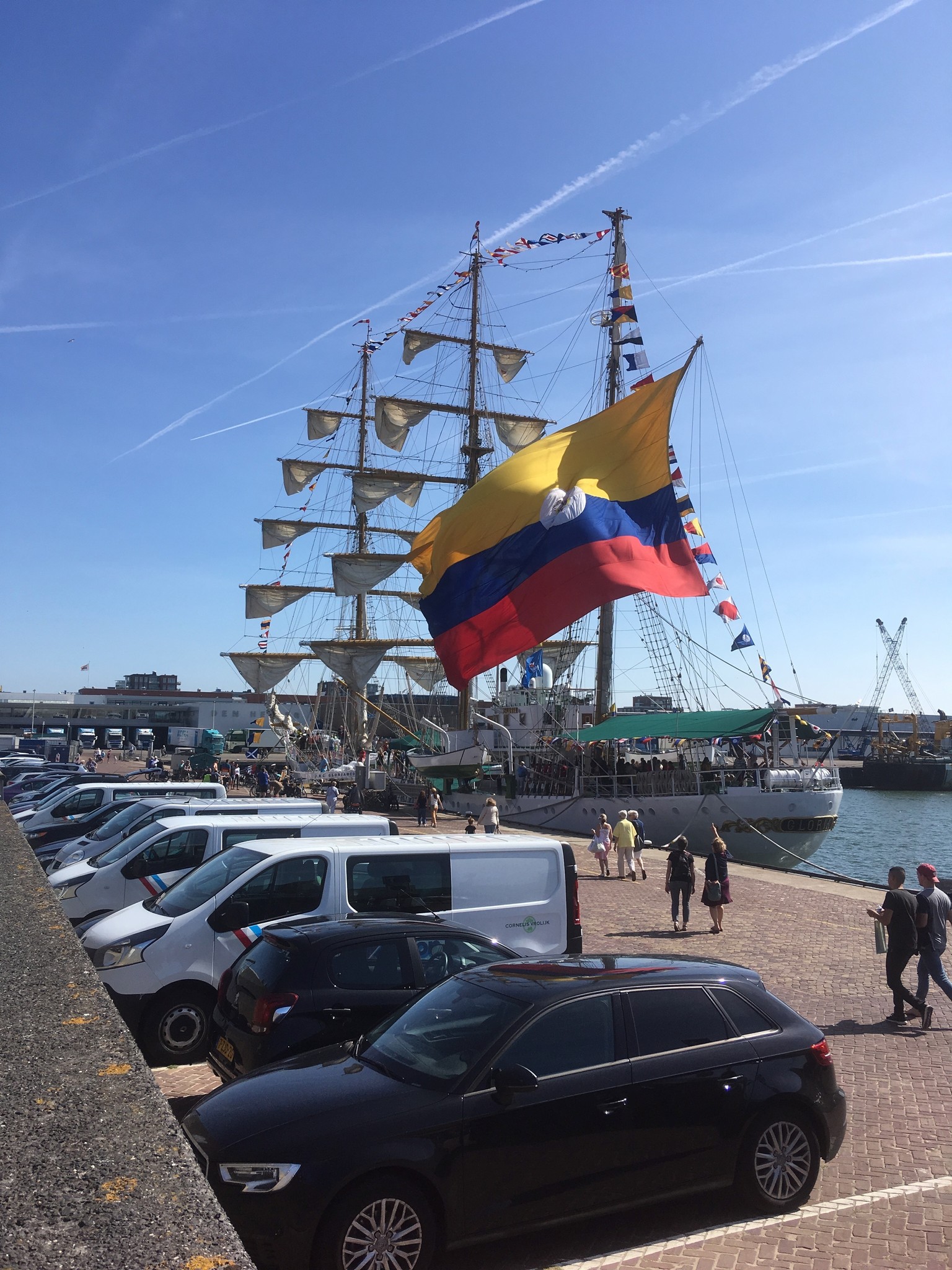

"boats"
[624,726,952,793]
[405,739,488,779]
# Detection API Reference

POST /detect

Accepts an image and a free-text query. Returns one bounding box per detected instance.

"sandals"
[674,922,687,931]
[709,926,723,933]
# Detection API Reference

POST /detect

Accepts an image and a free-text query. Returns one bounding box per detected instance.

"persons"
[93,748,112,764]
[701,823,733,932]
[590,751,823,794]
[517,761,528,795]
[904,863,952,1017]
[202,758,240,790]
[160,745,166,757]
[405,369,713,696]
[867,866,933,1028]
[79,757,98,773]
[148,756,164,782]
[113,755,118,764]
[255,764,285,798]
[129,745,134,756]
[325,781,339,813]
[591,810,647,880]
[412,788,443,828]
[665,836,696,932]
[290,728,404,778]
[178,760,192,781]
[477,797,499,835]
[465,818,477,834]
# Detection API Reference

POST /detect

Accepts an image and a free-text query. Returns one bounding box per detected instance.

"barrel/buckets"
[800,768,831,788]
[764,769,802,789]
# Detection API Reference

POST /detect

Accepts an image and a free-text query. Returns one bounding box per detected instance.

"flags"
[537,733,762,751]
[667,440,832,740]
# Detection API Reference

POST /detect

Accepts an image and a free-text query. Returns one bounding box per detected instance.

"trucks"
[46,727,64,734]
[77,728,98,750]
[104,728,125,750]
[223,728,286,754]
[167,726,225,755]
[20,728,37,735]
[135,728,155,751]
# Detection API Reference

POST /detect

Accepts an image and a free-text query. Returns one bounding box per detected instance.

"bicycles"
[238,769,399,815]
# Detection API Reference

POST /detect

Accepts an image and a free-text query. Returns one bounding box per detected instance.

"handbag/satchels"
[633,835,645,852]
[591,834,606,852]
[707,880,722,901]
[874,919,887,954]
[430,805,436,810]
[587,834,595,853]
[413,802,419,811]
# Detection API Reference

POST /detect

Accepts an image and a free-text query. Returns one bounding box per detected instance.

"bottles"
[875,904,883,912]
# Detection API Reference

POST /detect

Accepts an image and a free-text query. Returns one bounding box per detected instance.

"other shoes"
[626,873,632,878]
[642,869,647,880]
[632,871,637,881]
[431,823,433,826]
[606,868,610,876]
[424,824,426,827]
[432,826,437,828]
[617,876,626,879]
[417,824,421,827]
[598,873,604,877]
[905,1008,922,1018]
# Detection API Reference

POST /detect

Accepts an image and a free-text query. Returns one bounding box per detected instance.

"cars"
[179,945,849,1270]
[204,911,522,1086]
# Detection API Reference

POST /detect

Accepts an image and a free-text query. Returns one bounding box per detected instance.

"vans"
[72,829,583,1066]
[48,815,401,928]
[0,746,228,872]
[46,796,331,876]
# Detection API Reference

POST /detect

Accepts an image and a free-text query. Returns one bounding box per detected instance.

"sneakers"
[920,1004,933,1029]
[886,1013,908,1025]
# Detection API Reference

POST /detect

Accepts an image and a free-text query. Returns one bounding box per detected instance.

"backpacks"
[671,850,691,877]
[210,774,217,782]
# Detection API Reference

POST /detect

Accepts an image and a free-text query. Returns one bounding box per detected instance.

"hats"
[215,758,220,761]
[916,864,940,883]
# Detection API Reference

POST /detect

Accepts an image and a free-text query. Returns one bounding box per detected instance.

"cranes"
[836,617,908,759]
[875,618,935,752]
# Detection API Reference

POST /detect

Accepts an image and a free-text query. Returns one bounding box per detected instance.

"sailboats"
[204,209,869,869]
